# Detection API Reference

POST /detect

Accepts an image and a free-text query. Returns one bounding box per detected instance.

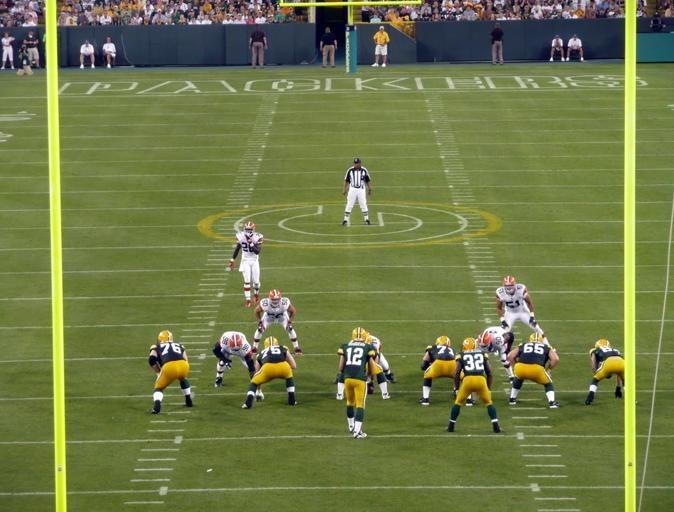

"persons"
[149,330,194,413]
[212,330,256,388]
[248,24,268,67]
[3,30,39,75]
[320,27,337,68]
[251,287,302,356]
[371,26,390,67]
[0,0,306,28]
[241,335,297,408]
[420,324,560,432]
[335,326,396,439]
[584,339,624,404]
[229,220,264,308]
[495,276,548,343]
[362,0,674,34]
[80,37,116,69]
[549,35,584,62]
[488,23,504,64]
[340,158,373,226]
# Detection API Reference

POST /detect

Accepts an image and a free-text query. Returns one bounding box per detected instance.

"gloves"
[230,259,235,272]
[285,321,293,331]
[502,321,508,328]
[529,317,536,327]
[258,321,264,332]
[546,368,553,378]
[245,235,252,243]
[453,387,458,396]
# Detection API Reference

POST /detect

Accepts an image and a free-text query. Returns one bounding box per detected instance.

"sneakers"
[292,401,297,405]
[241,393,264,410]
[152,401,192,413]
[509,376,517,405]
[464,398,474,407]
[336,393,345,400]
[295,349,302,355]
[343,220,347,225]
[418,397,429,405]
[348,425,367,438]
[445,426,503,433]
[252,348,257,354]
[366,220,369,224]
[215,382,222,388]
[585,392,623,405]
[245,293,259,308]
[381,377,396,400]
[550,401,560,409]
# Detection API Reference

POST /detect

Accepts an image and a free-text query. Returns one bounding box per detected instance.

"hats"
[353,158,359,162]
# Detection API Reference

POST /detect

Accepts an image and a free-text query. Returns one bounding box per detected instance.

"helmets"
[503,275,516,287]
[269,289,282,307]
[351,326,366,342]
[529,332,544,343]
[229,333,243,351]
[436,334,505,349]
[157,330,173,343]
[595,338,609,347]
[264,336,278,348]
[243,222,255,236]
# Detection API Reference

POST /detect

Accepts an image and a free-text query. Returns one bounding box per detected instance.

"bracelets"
[287,321,293,324]
[529,312,534,317]
[258,321,262,324]
[499,317,504,322]
